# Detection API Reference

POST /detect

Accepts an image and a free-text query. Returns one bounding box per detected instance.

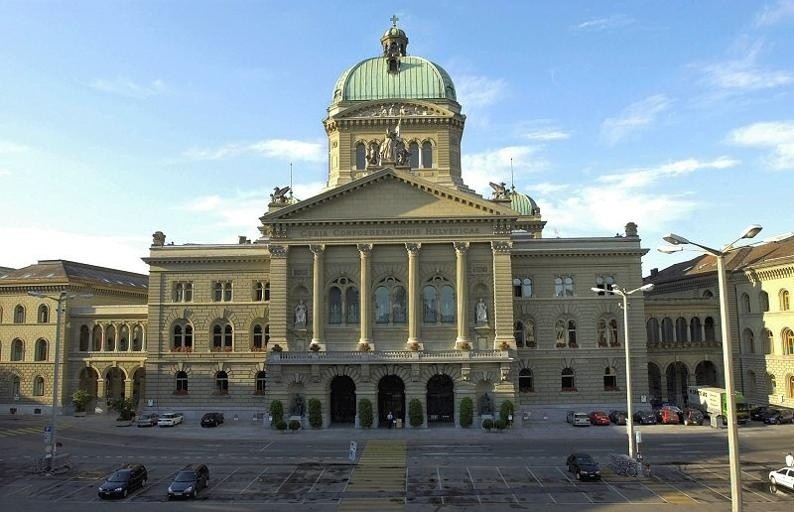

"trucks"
[686,385,751,425]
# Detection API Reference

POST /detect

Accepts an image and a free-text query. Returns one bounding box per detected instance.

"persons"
[294,299,307,328]
[476,297,487,321]
[785,451,794,467]
[387,412,394,429]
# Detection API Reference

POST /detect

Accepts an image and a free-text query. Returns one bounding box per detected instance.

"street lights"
[590,283,656,459]
[27,289,95,473]
[662,224,763,512]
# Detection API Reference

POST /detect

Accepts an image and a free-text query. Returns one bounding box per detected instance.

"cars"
[768,466,794,491]
[200,412,224,427]
[137,412,160,427]
[568,401,704,426]
[157,412,184,427]
[167,462,210,499]
[97,462,148,499]
[751,405,794,425]
[566,453,601,482]
